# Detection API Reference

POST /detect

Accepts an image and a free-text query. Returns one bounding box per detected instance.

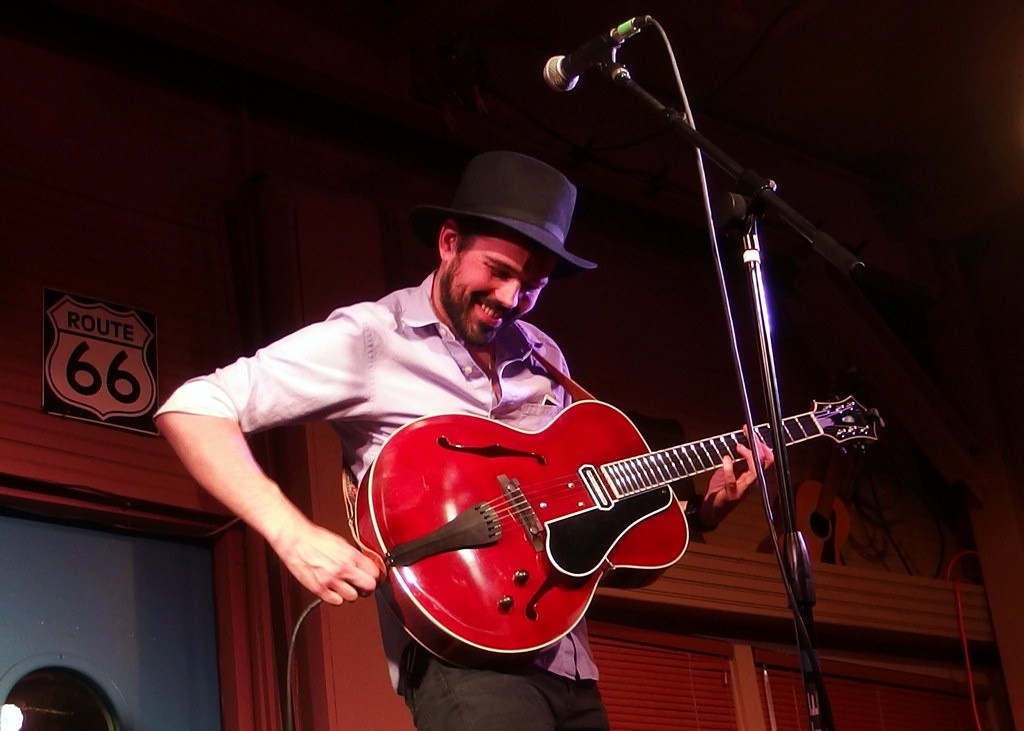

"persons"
[153,146,774,731]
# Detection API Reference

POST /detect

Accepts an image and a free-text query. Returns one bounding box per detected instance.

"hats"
[416,151,599,279]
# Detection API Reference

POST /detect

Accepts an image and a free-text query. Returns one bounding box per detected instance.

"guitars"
[353,392,885,667]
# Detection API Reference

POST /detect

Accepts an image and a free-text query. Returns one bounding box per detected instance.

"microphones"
[542,15,651,91]
